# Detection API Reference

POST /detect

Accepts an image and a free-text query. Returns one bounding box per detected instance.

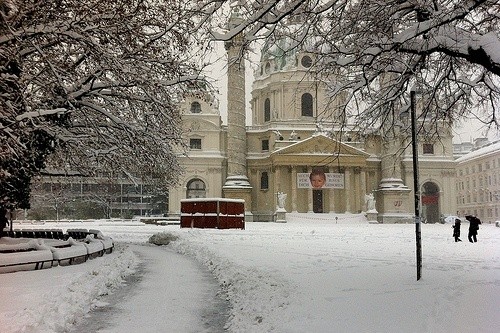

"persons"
[451,218,462,242]
[464,214,481,243]
[308,170,326,188]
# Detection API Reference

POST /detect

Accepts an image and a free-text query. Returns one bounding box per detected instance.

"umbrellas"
[464,215,481,225]
